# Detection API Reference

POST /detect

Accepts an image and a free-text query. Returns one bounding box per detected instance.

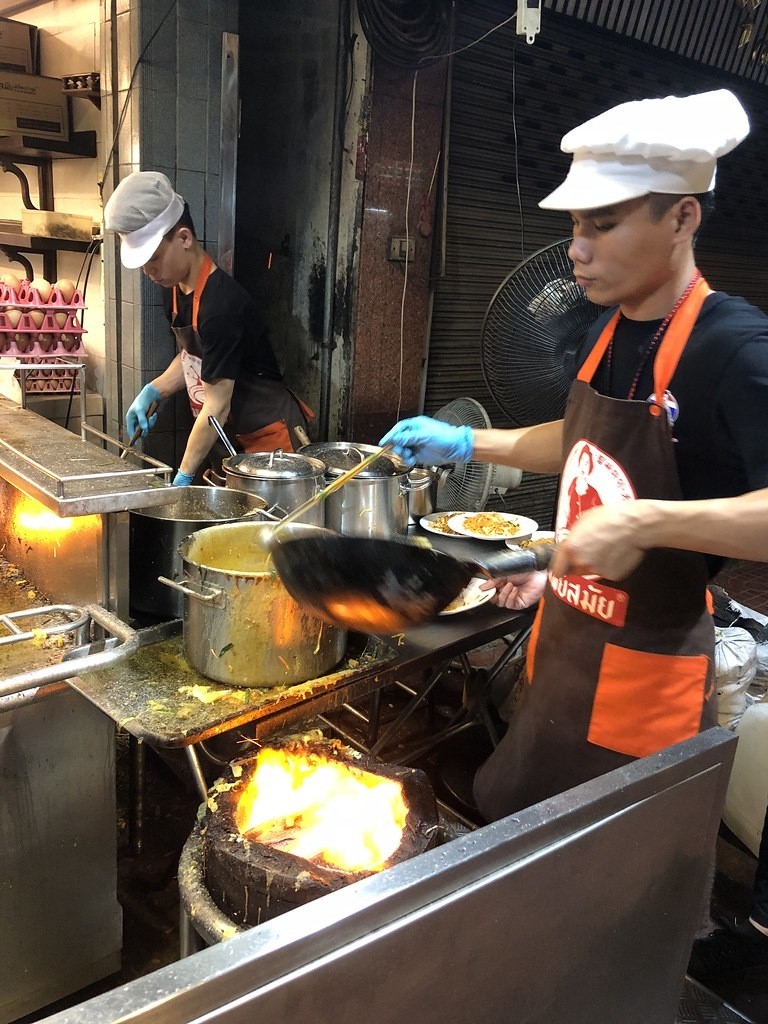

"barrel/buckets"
[722,703,768,858]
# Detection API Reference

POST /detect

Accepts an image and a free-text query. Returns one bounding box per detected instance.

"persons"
[379,88,768,820]
[103,171,311,485]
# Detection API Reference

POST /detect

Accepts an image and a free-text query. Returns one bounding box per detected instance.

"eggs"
[0,273,76,389]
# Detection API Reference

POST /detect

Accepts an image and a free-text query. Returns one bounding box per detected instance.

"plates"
[419,510,556,550]
[438,578,497,615]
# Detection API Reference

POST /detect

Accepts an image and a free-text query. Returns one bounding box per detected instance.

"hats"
[104,172,184,269]
[538,89,750,210]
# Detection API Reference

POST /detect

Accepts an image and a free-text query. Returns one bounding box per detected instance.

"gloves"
[125,384,162,446]
[379,414,475,467]
[172,469,195,486]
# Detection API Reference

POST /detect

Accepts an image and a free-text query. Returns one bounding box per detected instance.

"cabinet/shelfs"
[0,127,101,287]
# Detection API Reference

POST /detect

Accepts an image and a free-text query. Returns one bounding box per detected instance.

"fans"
[424,396,522,514]
[480,235,622,429]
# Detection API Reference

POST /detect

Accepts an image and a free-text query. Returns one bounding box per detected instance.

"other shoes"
[696,918,768,969]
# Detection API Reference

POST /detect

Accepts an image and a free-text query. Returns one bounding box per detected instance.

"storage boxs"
[21,208,93,244]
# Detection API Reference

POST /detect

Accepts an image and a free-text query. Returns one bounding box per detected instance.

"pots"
[267,537,565,635]
[157,521,361,690]
[124,438,434,620]
